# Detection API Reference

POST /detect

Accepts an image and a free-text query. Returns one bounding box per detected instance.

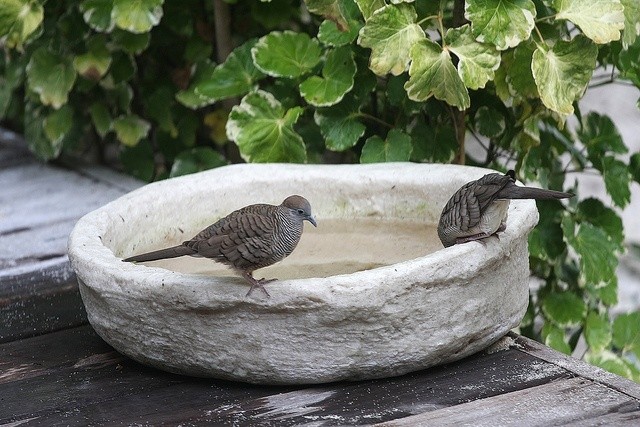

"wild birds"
[121,194,318,299]
[437,169,577,249]
